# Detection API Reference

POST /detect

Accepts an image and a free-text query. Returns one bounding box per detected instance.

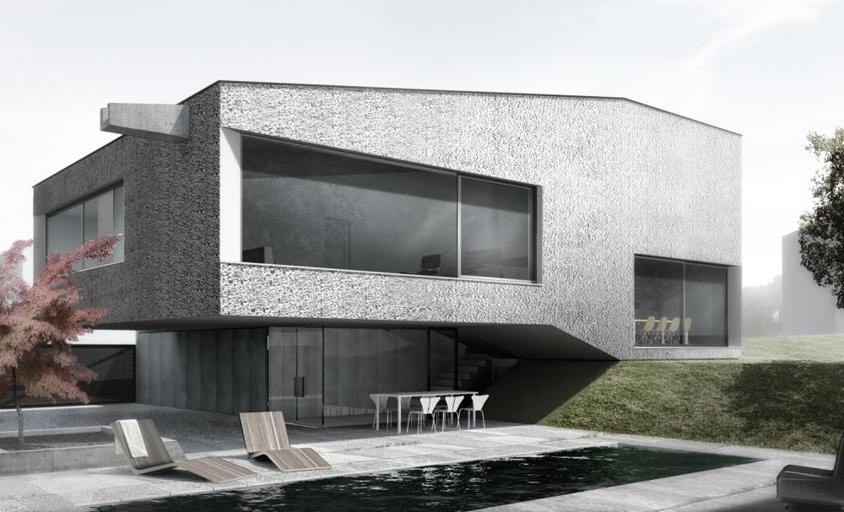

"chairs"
[636,315,692,346]
[775,433,844,512]
[110,418,257,483]
[240,411,331,472]
[368,390,490,434]
